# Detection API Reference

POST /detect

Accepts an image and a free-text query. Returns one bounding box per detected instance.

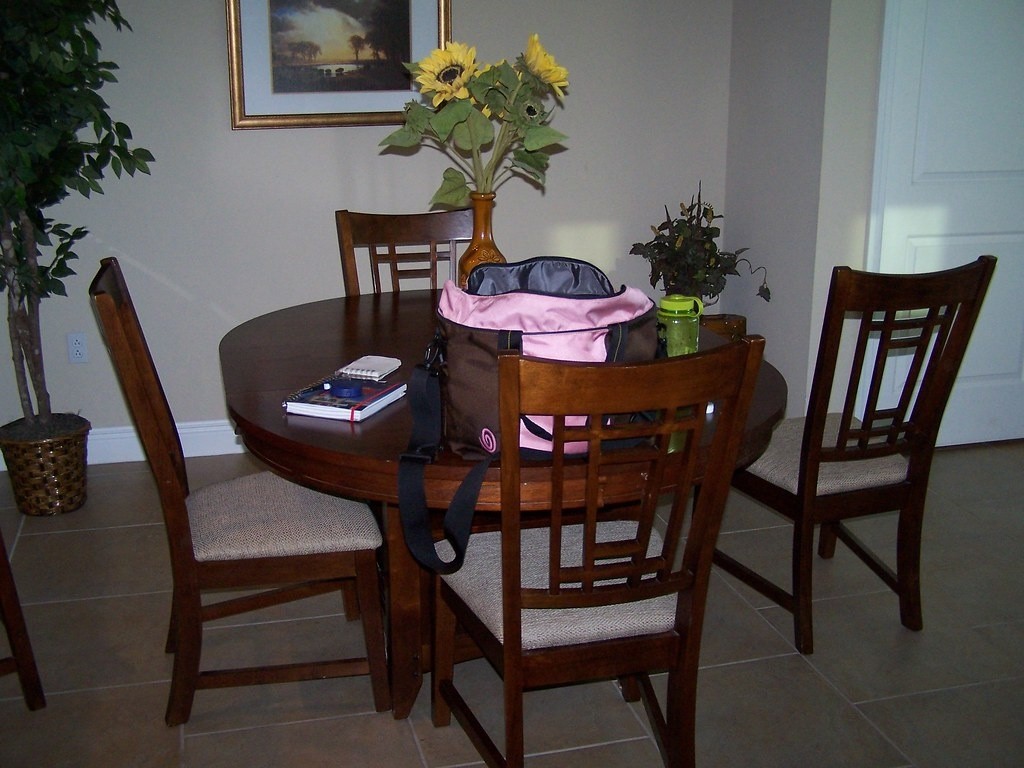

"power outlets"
[68,332,89,363]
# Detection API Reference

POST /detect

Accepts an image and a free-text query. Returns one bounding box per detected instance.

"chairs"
[430,335,766,768]
[691,254,1000,654]
[335,209,474,295]
[89,256,392,727]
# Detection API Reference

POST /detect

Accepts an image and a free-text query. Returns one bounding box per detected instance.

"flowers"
[379,33,568,207]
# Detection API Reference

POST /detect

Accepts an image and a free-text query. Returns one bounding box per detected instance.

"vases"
[458,192,508,291]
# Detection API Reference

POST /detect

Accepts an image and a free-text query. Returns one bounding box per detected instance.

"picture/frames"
[223,0,451,130]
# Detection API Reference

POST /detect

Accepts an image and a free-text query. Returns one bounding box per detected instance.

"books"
[343,355,401,377]
[282,374,407,422]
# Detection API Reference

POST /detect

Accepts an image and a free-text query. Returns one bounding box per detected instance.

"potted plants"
[629,178,772,336]
[0,0,156,518]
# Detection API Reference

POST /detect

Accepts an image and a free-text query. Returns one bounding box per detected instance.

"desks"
[219,289,787,723]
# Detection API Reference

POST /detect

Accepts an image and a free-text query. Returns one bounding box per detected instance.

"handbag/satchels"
[433,253,660,463]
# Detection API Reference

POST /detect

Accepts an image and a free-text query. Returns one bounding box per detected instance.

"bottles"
[655,295,704,419]
